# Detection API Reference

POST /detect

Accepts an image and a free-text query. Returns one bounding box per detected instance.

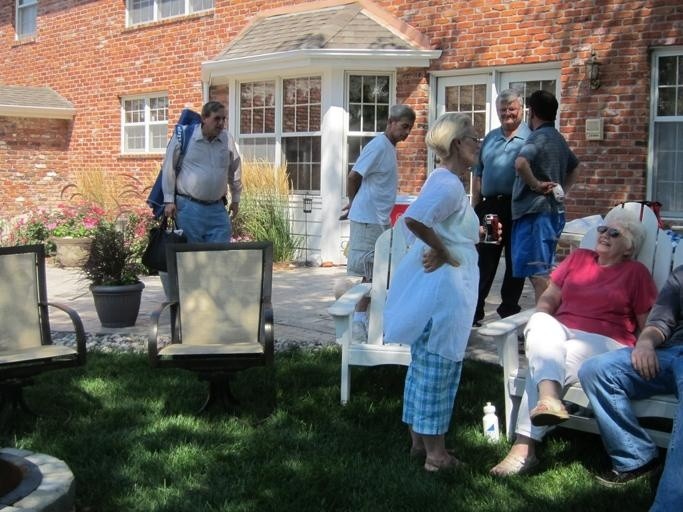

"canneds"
[483,214,498,244]
[551,182,565,201]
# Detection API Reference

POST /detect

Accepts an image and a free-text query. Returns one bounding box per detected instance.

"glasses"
[465,133,480,143]
[596,225,629,240]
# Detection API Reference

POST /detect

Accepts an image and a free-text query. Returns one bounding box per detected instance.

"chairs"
[147,240,274,416]
[328,226,412,408]
[0,243,86,438]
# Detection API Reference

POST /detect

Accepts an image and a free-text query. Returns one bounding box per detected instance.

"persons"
[578,264,681,510]
[161,101,242,244]
[510,90,579,308]
[384,112,482,472]
[491,209,658,480]
[337,104,416,325]
[469,89,532,323]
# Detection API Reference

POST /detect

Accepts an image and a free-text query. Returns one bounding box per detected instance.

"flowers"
[46,201,106,238]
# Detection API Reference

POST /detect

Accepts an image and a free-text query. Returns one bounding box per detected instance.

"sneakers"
[352,320,368,344]
[595,457,662,489]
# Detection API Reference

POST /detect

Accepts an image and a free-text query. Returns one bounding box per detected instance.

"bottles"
[481,401,500,445]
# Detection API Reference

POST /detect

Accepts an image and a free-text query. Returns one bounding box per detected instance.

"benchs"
[477,200,682,450]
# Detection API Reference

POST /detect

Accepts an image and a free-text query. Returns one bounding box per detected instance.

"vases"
[51,235,94,268]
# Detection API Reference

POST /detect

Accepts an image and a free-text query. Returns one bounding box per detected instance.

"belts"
[183,194,218,205]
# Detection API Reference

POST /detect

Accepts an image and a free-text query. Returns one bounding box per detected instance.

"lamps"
[583,48,601,89]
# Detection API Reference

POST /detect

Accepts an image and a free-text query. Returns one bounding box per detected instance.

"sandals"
[410,396,571,477]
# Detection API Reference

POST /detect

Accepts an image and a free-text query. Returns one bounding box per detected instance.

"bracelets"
[529,181,539,192]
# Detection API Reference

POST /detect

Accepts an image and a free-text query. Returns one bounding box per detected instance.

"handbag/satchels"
[142,227,187,273]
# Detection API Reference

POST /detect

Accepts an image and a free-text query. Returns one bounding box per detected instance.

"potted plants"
[77,224,149,327]
